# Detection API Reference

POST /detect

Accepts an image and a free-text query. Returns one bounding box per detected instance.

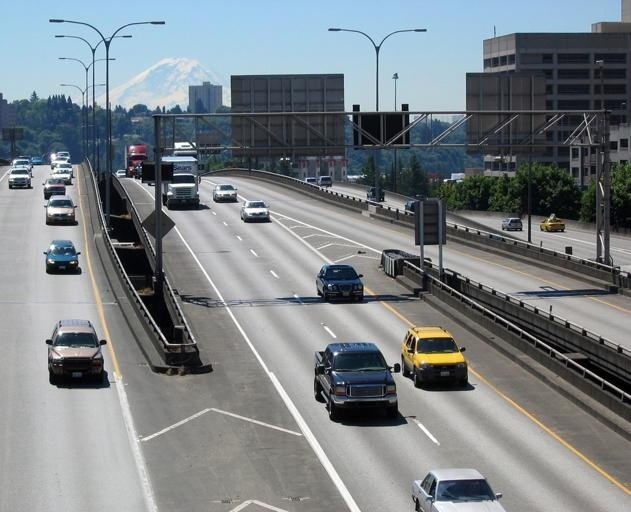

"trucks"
[116,138,201,209]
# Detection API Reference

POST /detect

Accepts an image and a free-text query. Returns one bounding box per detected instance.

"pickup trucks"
[314,340,400,421]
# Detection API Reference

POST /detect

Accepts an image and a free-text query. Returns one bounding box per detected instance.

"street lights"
[329,24,428,111]
[392,70,399,110]
[59,82,106,157]
[49,15,169,230]
[58,56,116,171]
[54,34,134,182]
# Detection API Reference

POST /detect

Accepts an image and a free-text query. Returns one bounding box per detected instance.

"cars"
[315,260,368,300]
[213,183,238,203]
[3,145,73,195]
[540,214,565,232]
[406,196,427,215]
[403,325,469,389]
[43,238,81,273]
[306,177,318,185]
[409,467,506,507]
[366,187,386,201]
[45,317,108,382]
[239,196,267,223]
[319,176,333,187]
[44,194,78,223]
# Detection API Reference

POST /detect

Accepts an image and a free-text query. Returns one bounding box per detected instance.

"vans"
[501,217,524,231]
